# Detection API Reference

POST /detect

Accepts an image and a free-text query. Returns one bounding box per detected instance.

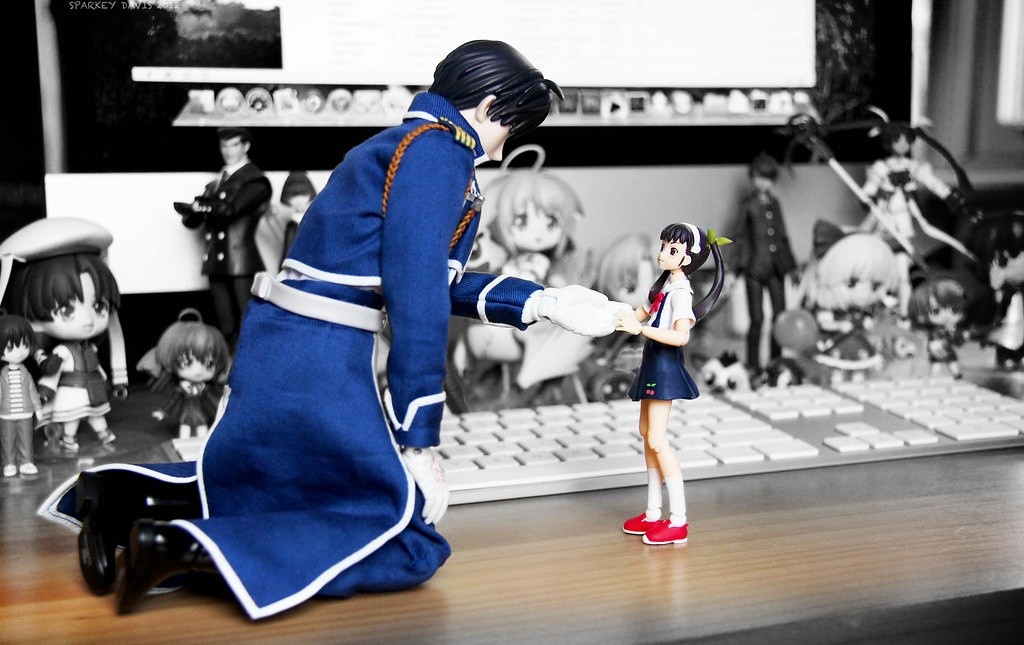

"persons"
[613,222,731,545]
[173,126,273,356]
[280,173,315,272]
[478,105,1024,396]
[34,39,632,620]
[1,217,129,477]
[138,310,230,444]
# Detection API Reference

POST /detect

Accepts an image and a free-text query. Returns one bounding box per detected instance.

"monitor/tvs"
[32,0,934,293]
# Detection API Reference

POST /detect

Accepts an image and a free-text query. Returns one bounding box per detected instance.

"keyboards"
[435,376,1024,505]
